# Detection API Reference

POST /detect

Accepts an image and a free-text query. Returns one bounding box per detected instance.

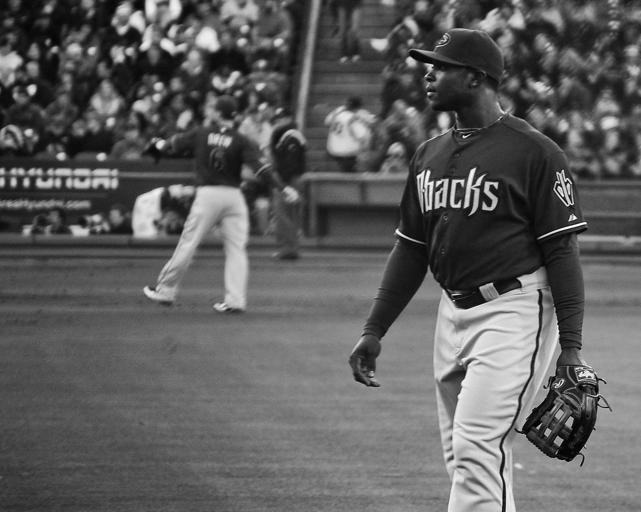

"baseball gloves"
[522,365,600,462]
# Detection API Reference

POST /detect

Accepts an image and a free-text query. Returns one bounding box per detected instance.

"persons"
[347,26,586,511]
[136,89,300,313]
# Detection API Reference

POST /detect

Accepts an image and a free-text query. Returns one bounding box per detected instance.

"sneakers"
[212,300,238,313]
[142,283,177,306]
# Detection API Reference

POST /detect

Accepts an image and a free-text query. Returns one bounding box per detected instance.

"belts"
[439,275,525,310]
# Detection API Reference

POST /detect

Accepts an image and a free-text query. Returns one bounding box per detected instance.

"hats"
[270,107,294,122]
[206,94,238,117]
[404,27,507,85]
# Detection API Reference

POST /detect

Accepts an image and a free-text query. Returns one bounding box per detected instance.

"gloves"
[282,186,302,205]
